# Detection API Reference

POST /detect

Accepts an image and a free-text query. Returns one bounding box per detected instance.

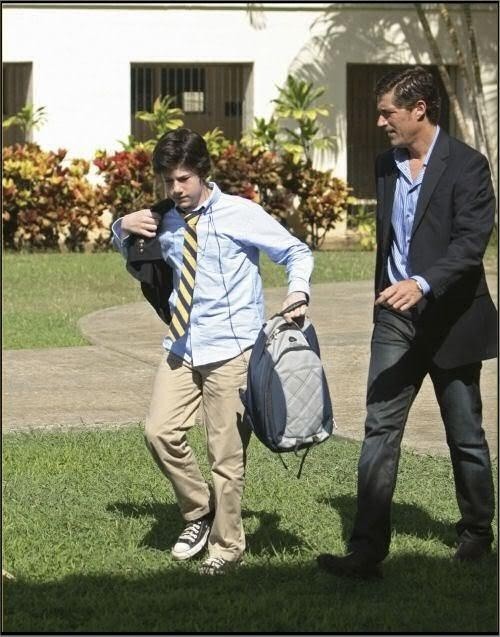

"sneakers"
[172,512,243,577]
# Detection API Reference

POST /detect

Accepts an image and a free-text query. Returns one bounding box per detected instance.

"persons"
[334,68,499,584]
[109,127,316,575]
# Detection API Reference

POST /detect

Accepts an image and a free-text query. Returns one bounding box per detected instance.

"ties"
[167,206,204,343]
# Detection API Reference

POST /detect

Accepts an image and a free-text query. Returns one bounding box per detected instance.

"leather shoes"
[317,553,384,581]
[450,537,492,559]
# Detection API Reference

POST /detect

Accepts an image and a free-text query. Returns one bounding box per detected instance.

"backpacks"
[240,312,334,453]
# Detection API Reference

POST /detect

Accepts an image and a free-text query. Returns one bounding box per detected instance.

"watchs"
[417,280,423,292]
